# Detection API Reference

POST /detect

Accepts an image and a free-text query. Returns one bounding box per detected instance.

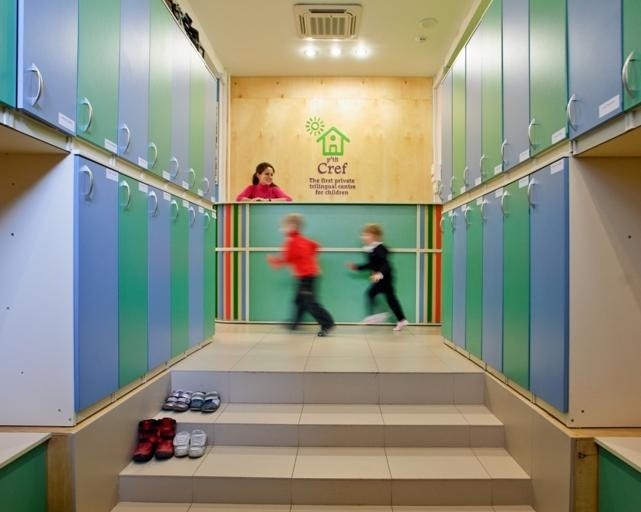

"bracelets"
[269,198,271,202]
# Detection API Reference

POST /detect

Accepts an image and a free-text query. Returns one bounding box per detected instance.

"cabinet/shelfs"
[440,0,641,512]
[0,0,218,512]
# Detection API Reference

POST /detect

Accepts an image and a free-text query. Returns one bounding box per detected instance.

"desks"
[212,202,443,326]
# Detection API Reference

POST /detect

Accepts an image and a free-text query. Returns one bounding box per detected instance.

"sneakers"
[162,389,221,413]
[133,417,207,463]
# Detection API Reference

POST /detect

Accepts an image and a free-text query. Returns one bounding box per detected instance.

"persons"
[235,162,292,202]
[266,212,335,336]
[346,224,408,331]
